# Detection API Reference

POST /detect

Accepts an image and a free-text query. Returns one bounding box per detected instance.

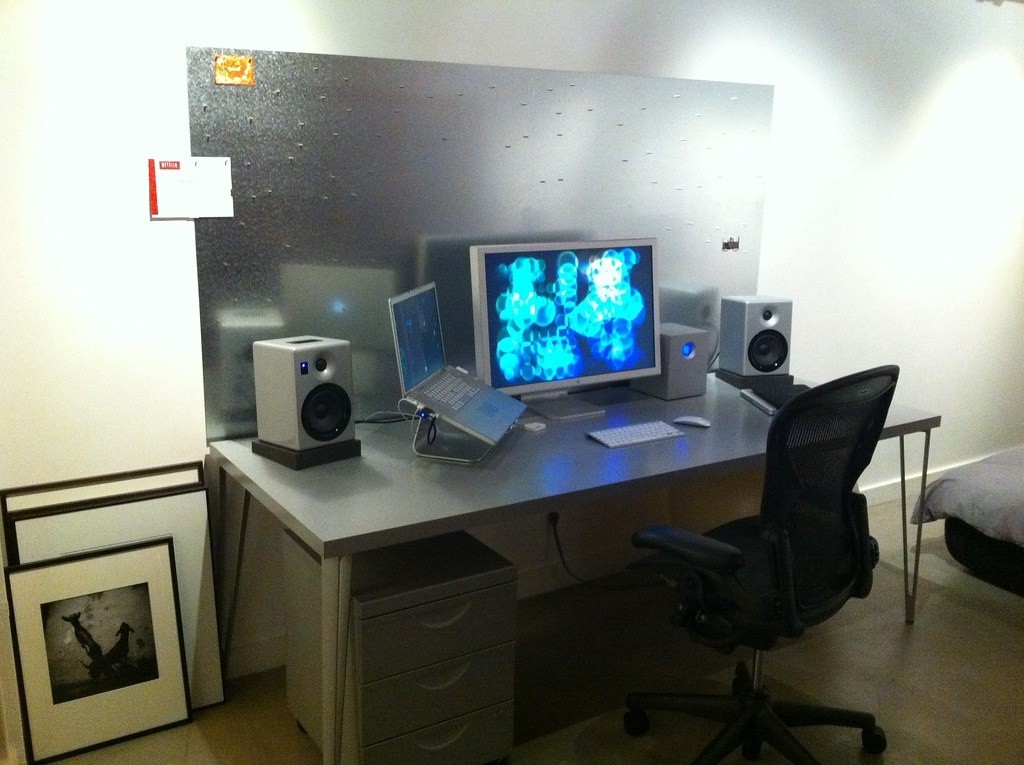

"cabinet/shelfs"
[281,527,515,765]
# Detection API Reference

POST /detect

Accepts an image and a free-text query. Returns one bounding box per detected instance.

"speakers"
[252,336,355,451]
[721,295,792,376]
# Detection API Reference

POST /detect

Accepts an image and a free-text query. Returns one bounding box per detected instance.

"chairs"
[623,364,900,765]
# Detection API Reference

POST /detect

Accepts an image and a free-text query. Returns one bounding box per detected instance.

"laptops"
[388,281,528,445]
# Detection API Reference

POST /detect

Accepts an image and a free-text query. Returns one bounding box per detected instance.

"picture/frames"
[0,460,204,514]
[3,487,226,713]
[4,534,192,765]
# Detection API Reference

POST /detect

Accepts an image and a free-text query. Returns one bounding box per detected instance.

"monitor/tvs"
[468,238,662,421]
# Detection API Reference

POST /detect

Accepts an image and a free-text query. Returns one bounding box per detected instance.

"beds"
[909,442,1024,599]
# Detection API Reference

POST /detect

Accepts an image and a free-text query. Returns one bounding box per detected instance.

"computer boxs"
[629,322,710,401]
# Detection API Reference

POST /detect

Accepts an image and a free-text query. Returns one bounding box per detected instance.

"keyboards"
[584,420,686,449]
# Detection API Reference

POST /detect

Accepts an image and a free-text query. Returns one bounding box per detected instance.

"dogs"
[62,612,102,660]
[78,621,135,680]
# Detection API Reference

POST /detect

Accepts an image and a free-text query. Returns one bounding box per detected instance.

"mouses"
[673,415,711,427]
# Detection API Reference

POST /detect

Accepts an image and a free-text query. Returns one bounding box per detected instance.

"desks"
[210,372,940,765]
